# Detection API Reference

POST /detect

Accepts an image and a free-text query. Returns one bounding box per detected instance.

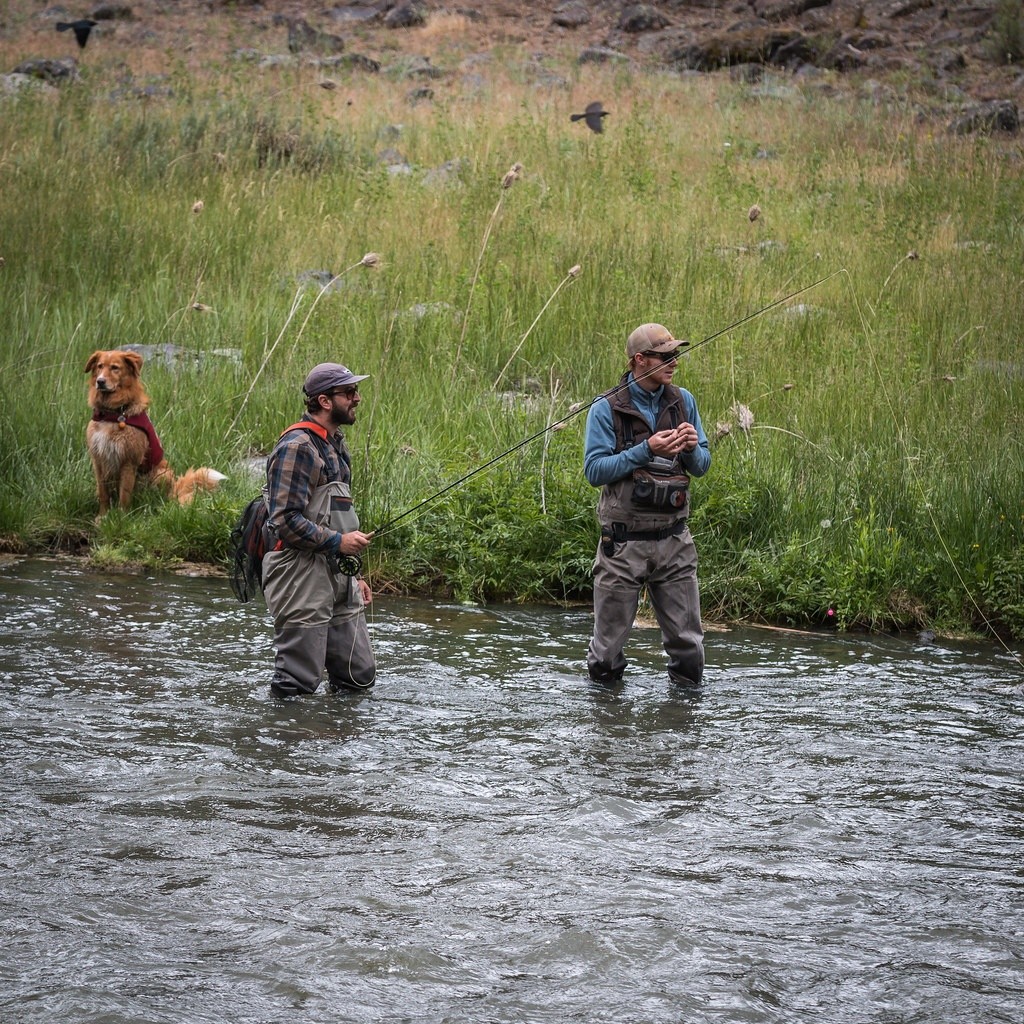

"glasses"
[642,350,680,363]
[327,386,359,400]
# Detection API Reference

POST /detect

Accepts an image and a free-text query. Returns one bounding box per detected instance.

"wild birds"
[571,102,611,135]
[56,18,101,49]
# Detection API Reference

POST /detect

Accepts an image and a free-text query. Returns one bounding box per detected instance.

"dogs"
[85,350,227,524]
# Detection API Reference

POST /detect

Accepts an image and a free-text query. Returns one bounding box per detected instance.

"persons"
[261,362,376,698]
[583,322,712,684]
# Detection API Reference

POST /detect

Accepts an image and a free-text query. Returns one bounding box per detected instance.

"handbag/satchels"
[226,495,271,605]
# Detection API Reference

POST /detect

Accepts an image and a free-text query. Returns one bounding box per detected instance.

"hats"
[303,363,370,396]
[626,323,690,359]
